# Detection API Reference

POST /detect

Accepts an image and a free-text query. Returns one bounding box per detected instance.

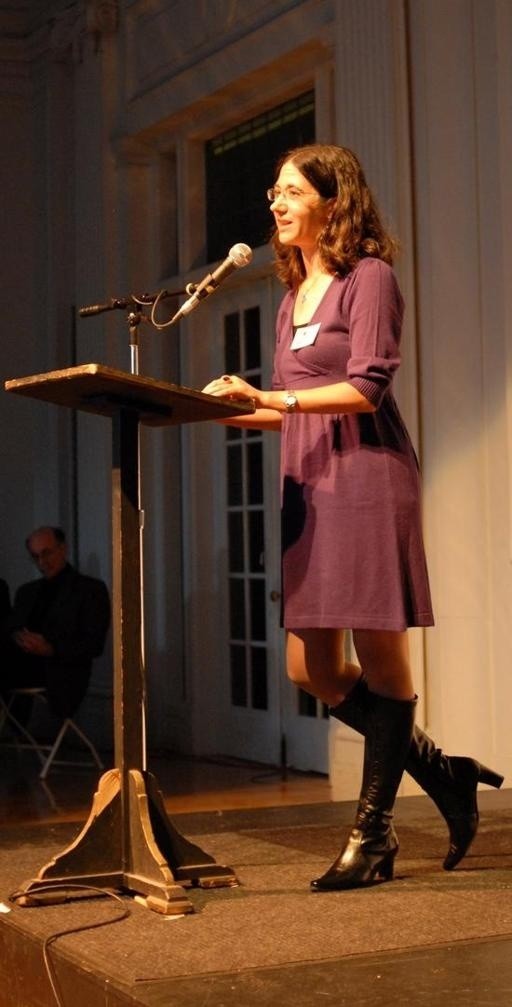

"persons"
[1,527,114,788]
[196,142,506,893]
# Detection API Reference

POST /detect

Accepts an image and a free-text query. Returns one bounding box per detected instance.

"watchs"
[283,389,299,412]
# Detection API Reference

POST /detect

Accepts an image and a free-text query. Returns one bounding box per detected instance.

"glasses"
[267,187,318,201]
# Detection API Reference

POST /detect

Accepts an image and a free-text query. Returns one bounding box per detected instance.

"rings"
[221,375,232,384]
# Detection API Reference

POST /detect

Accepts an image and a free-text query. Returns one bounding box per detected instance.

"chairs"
[1,687,105,779]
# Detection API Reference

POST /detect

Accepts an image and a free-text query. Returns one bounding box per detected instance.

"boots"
[308,693,418,891]
[327,668,505,871]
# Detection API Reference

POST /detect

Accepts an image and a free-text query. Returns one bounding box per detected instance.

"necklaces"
[297,266,326,303]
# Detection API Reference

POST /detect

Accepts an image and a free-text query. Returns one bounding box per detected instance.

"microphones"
[171,243,254,323]
